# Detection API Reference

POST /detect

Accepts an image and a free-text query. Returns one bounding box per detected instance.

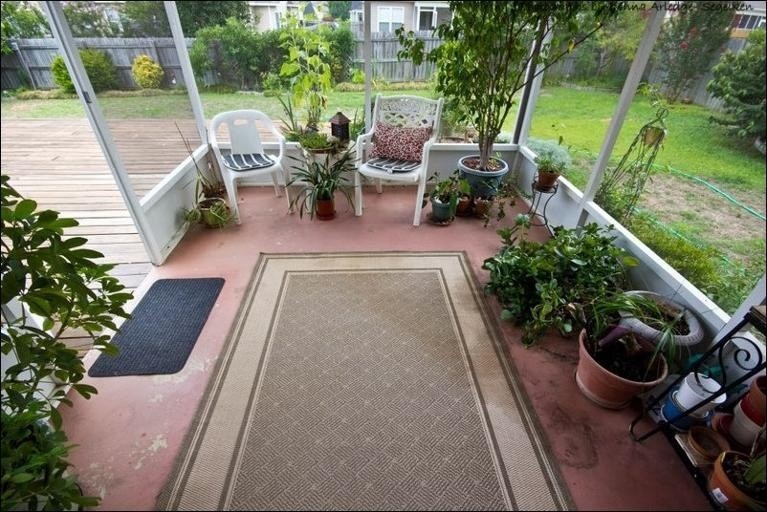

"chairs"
[355,92,445,226]
[208,108,295,227]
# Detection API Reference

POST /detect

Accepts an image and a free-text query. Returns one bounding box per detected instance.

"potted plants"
[395,0,627,227]
[583,281,705,381]
[282,141,369,222]
[706,449,767,511]
[183,176,239,232]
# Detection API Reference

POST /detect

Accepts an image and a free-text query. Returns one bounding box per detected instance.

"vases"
[657,364,767,465]
[574,324,670,410]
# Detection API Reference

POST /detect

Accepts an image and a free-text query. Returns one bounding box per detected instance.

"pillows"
[370,120,433,162]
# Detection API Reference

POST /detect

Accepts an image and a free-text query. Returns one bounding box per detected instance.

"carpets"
[87,278,226,377]
[153,252,578,511]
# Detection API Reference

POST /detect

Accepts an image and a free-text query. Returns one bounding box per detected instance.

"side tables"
[302,140,358,215]
